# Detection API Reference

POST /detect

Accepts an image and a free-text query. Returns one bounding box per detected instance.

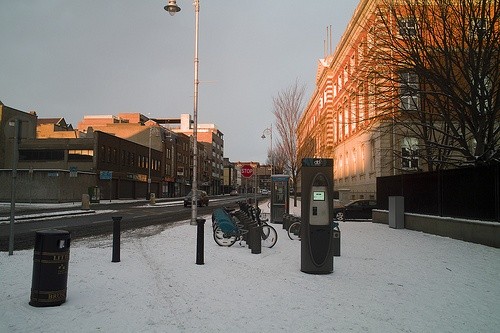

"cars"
[262,190,268,195]
[333,199,377,221]
[184,190,209,207]
[230,190,238,196]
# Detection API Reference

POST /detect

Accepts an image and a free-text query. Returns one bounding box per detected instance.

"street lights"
[148,128,160,194]
[164,1,199,226]
[261,124,272,176]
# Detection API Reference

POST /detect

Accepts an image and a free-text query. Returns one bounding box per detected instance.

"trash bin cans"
[29,229,71,307]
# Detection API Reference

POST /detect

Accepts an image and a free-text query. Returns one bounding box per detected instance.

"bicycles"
[211,202,278,248]
[287,218,302,241]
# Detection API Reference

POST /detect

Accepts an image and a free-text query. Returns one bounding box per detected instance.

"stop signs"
[241,165,253,178]
